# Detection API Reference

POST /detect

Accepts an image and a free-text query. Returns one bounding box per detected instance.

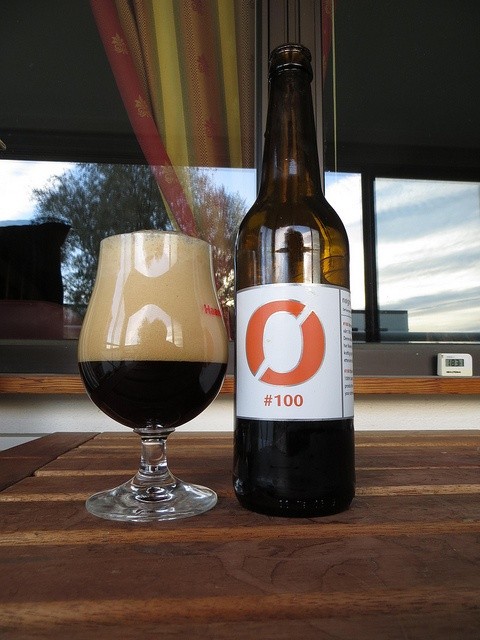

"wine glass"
[78,233,229,521]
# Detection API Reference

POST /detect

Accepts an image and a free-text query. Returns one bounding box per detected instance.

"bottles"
[233,44,356,517]
